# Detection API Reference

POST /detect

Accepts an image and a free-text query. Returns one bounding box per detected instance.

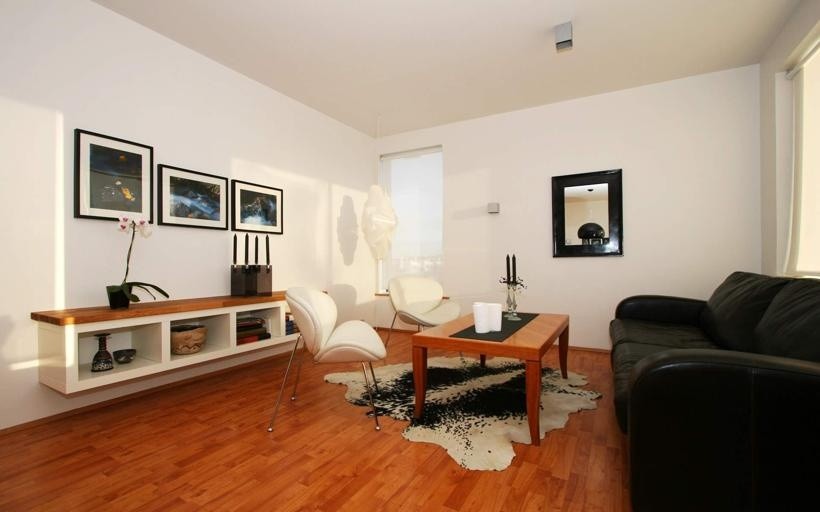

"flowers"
[110,214,169,304]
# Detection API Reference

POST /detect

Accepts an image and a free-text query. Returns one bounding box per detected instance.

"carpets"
[324,356,602,472]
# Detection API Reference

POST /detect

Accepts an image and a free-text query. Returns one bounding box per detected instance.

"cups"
[473,301,503,334]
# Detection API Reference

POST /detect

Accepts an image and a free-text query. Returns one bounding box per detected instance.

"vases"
[108,286,132,309]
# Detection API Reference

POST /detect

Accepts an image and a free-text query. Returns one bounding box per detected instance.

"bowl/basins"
[113,348,138,364]
[170,324,210,355]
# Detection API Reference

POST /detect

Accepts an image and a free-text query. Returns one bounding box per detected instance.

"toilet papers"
[472,302,502,334]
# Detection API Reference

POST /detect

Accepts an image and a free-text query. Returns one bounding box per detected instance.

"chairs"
[386,275,463,359]
[267,288,386,432]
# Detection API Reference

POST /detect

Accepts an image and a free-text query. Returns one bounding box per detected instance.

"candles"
[245,233,249,266]
[506,255,511,281]
[511,253,517,283]
[256,236,258,265]
[266,235,270,266]
[233,233,237,266]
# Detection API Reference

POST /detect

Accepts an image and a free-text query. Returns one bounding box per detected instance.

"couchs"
[608,271,820,511]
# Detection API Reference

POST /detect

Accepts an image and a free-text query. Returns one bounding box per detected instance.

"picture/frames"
[551,168,623,257]
[231,179,283,236]
[74,128,154,224]
[157,164,228,232]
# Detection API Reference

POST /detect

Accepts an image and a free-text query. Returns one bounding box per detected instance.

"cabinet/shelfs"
[30,292,296,398]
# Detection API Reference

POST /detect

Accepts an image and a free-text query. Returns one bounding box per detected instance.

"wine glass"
[91,332,114,372]
